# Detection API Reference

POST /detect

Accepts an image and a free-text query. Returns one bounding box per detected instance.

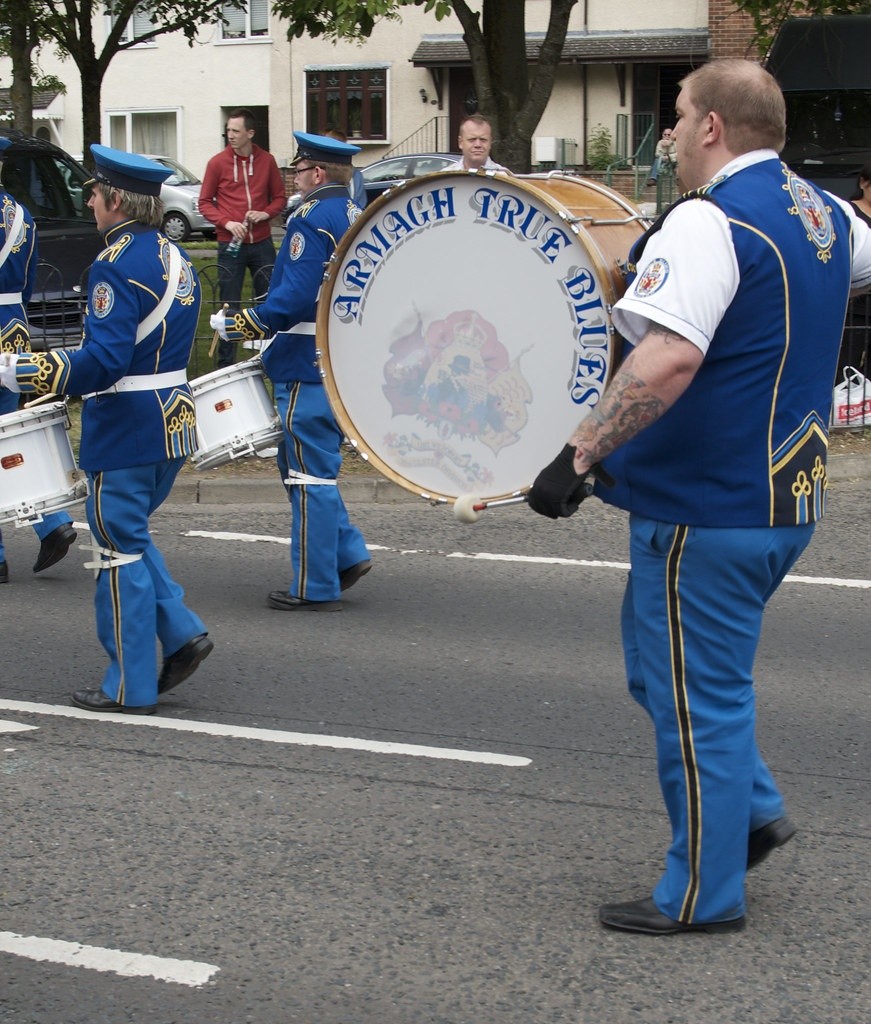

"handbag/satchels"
[829,366,871,429]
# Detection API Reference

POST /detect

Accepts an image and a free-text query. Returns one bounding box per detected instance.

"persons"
[0,136,75,582]
[324,128,367,208]
[0,144,212,715]
[440,115,510,174]
[210,132,372,611]
[524,60,871,933]
[647,128,677,186]
[198,108,286,369]
[848,162,871,228]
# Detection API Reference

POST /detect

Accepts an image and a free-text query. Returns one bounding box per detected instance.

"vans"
[764,13,871,201]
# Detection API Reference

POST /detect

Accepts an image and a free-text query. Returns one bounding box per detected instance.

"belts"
[277,321,316,336]
[0,292,23,305]
[80,368,189,401]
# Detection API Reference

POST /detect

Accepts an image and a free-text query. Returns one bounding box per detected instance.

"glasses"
[663,134,670,136]
[293,165,327,177]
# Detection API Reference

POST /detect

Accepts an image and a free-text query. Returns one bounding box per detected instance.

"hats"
[289,130,363,166]
[82,144,175,196]
[0,135,13,151]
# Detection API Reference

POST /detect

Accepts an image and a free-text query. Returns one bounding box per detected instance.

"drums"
[185,361,282,470]
[317,168,662,516]
[0,398,88,527]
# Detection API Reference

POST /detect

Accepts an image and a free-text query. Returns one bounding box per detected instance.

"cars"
[55,153,217,243]
[0,127,107,352]
[281,153,462,230]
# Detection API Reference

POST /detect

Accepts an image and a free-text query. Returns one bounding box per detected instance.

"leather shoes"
[33,522,78,572]
[339,560,373,592]
[69,688,159,716]
[747,815,800,870]
[598,895,747,936]
[267,590,345,612]
[157,632,214,694]
[0,559,9,583]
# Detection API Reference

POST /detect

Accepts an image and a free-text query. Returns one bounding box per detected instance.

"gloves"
[527,442,594,519]
[210,309,230,342]
[0,352,21,394]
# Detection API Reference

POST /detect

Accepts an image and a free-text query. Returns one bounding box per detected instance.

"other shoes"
[646,179,656,186]
